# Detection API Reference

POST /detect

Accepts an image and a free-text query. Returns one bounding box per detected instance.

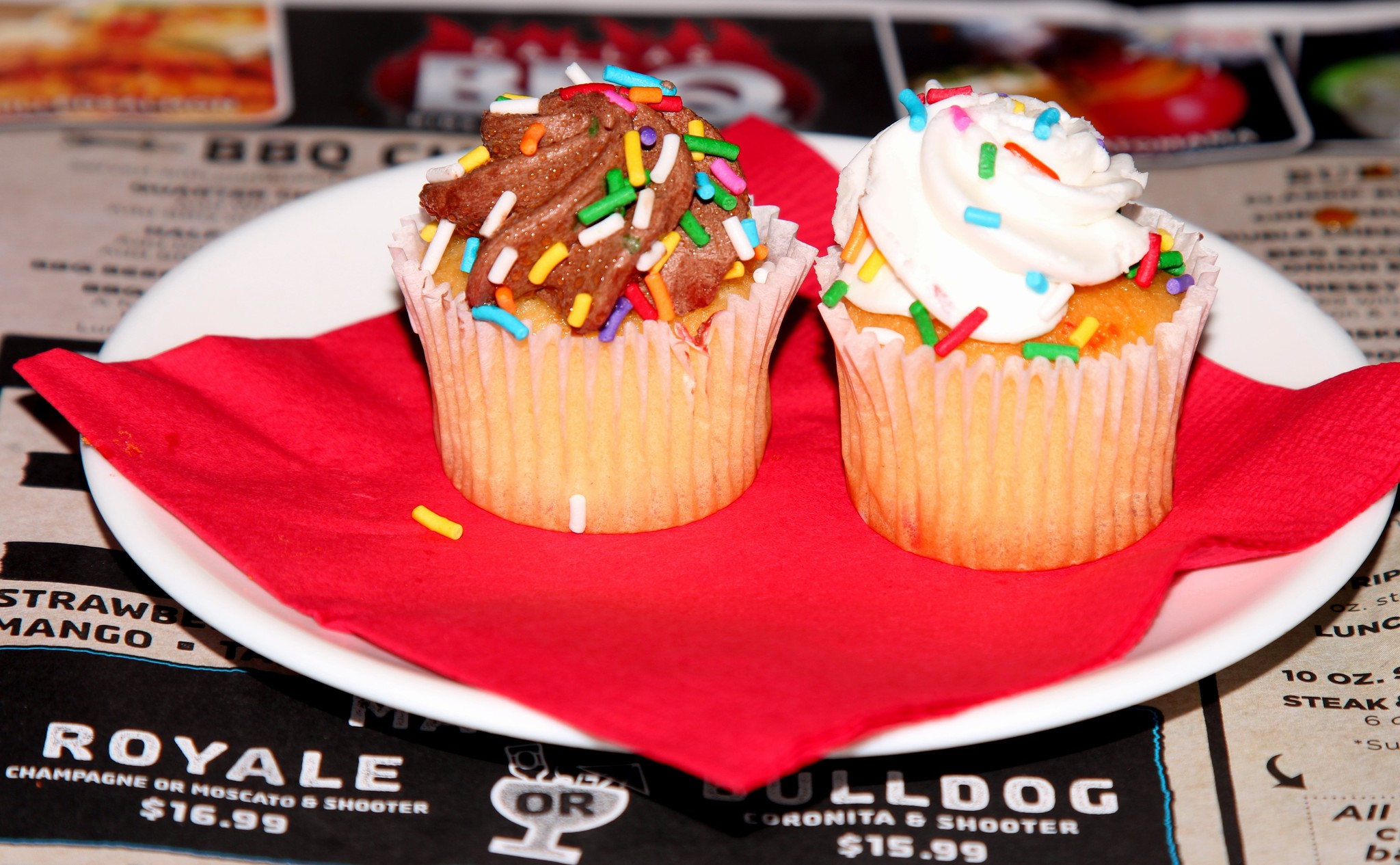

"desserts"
[813,79,1218,570]
[385,62,820,532]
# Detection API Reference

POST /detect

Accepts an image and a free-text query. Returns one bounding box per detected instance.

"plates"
[78,130,1400,759]
